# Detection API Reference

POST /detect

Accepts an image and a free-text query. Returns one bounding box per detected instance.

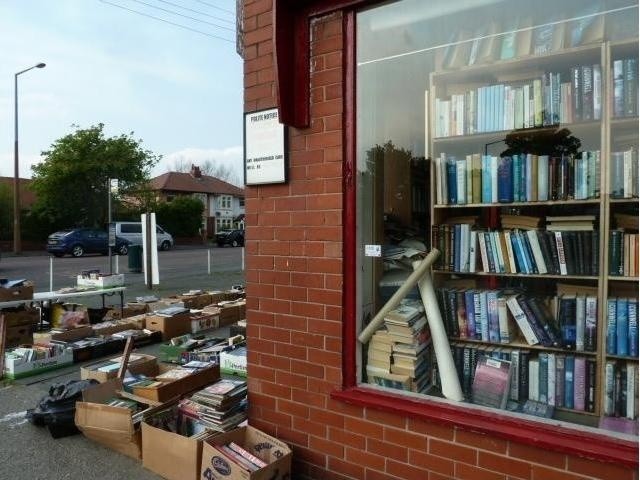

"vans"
[103,221,174,251]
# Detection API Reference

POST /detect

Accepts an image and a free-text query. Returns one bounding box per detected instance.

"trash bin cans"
[128,245,143,272]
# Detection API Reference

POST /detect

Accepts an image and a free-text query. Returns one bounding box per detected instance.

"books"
[448,339,639,421]
[436,57,639,137]
[366,298,433,393]
[435,147,639,205]
[3,268,269,471]
[435,286,640,358]
[430,212,639,277]
[439,5,607,69]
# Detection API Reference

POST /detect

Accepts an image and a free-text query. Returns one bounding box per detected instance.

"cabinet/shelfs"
[422,48,604,412]
[606,37,639,440]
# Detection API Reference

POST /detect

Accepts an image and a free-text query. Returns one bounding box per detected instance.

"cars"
[46,227,132,256]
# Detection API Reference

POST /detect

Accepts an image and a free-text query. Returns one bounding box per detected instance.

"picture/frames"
[243,108,289,187]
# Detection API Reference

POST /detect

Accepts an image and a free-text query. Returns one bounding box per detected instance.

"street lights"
[13,63,46,253]
[484,135,521,176]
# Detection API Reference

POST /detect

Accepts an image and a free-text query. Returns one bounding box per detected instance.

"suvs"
[214,229,244,247]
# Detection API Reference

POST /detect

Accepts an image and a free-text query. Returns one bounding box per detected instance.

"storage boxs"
[204,424,290,480]
[142,407,228,480]
